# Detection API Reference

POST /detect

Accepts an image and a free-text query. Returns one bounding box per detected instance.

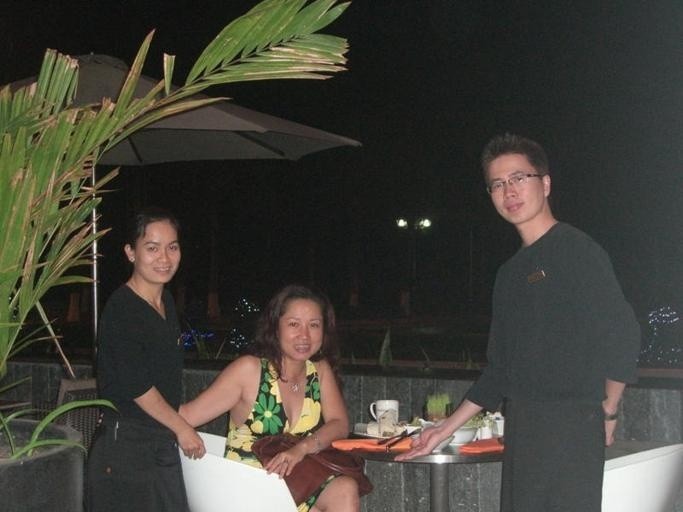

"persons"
[81,208,207,511]
[394,134,640,511]
[177,283,362,512]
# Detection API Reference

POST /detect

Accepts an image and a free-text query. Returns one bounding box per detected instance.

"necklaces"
[281,373,302,392]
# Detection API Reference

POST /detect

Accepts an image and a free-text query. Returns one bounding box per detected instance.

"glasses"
[487,171,540,195]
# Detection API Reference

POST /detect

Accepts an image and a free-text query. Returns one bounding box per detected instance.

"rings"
[282,459,288,464]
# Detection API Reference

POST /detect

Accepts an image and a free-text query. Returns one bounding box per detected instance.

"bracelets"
[309,432,320,453]
[603,412,618,421]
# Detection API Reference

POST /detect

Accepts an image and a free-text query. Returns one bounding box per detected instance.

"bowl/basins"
[418,418,478,446]
[411,434,455,453]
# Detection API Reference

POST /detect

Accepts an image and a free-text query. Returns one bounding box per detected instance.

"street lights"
[392,216,433,359]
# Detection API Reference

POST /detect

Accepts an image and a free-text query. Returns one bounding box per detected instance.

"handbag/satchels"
[251,432,374,506]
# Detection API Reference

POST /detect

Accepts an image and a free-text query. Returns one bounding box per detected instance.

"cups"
[369,400,400,425]
[483,418,504,440]
[476,420,493,440]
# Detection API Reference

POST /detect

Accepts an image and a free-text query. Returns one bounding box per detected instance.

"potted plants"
[0,0,354,511]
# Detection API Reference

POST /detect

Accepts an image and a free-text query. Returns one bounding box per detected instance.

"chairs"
[29,379,100,449]
[602,441,683,512]
[177,431,300,512]
